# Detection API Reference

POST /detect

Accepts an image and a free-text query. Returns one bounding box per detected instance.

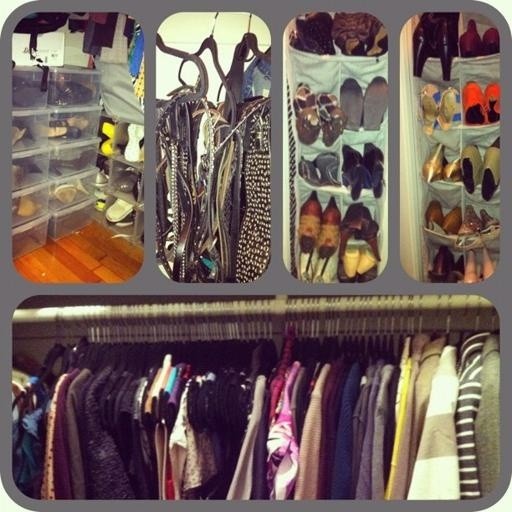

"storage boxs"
[11,31,104,262]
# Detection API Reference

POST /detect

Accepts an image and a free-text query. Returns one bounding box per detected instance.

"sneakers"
[419,82,501,284]
[293,78,390,282]
[93,113,146,229]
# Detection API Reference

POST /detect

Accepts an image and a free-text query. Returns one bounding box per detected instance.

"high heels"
[411,14,459,81]
[52,180,91,204]
[50,150,95,175]
[16,196,43,217]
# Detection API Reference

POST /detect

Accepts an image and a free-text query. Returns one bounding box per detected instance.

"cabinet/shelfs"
[95,111,145,248]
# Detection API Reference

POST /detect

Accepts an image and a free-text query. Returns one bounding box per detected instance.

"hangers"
[154,10,273,137]
[12,291,501,365]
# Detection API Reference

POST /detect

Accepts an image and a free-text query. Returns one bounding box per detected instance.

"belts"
[152,88,243,284]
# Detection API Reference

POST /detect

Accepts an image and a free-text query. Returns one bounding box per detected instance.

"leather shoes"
[460,21,481,57]
[483,26,499,52]
[50,113,90,139]
